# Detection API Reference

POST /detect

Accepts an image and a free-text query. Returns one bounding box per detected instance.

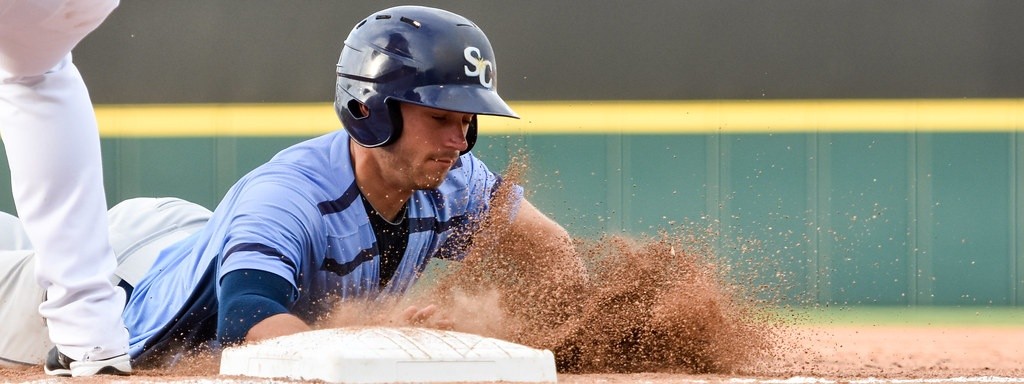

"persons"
[0,4,589,369]
[0,0,136,378]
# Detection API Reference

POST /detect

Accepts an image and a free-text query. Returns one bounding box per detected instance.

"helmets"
[333,5,521,156]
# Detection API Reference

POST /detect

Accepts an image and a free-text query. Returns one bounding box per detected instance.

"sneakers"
[44,345,133,377]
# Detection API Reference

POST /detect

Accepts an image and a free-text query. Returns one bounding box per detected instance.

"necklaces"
[359,181,408,225]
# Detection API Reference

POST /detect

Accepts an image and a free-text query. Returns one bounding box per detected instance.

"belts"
[117,279,133,308]
